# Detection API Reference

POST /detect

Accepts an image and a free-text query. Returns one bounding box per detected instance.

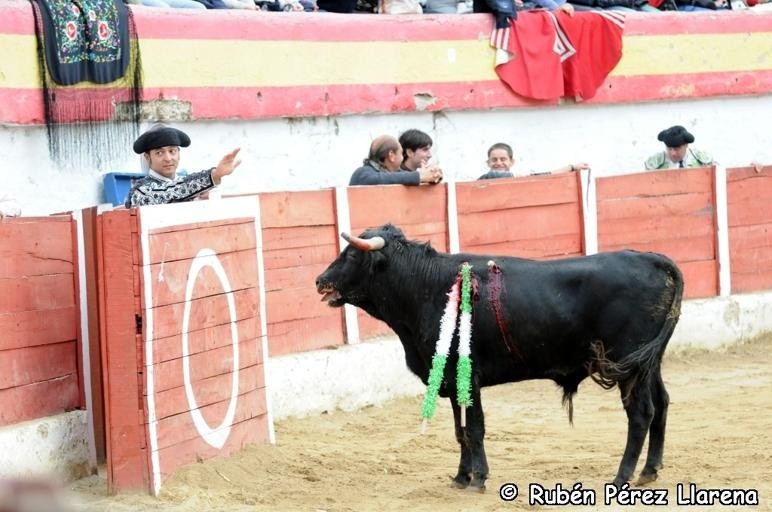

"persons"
[475,141,590,181]
[125,2,772,21]
[397,127,434,172]
[124,123,243,206]
[643,124,765,175]
[347,133,447,187]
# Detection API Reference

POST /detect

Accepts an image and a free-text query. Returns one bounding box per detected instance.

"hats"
[132,120,192,154]
[657,124,695,148]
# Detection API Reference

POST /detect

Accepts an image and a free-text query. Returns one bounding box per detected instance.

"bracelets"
[567,163,575,172]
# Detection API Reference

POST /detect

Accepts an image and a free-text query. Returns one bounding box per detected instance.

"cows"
[314,222,687,503]
